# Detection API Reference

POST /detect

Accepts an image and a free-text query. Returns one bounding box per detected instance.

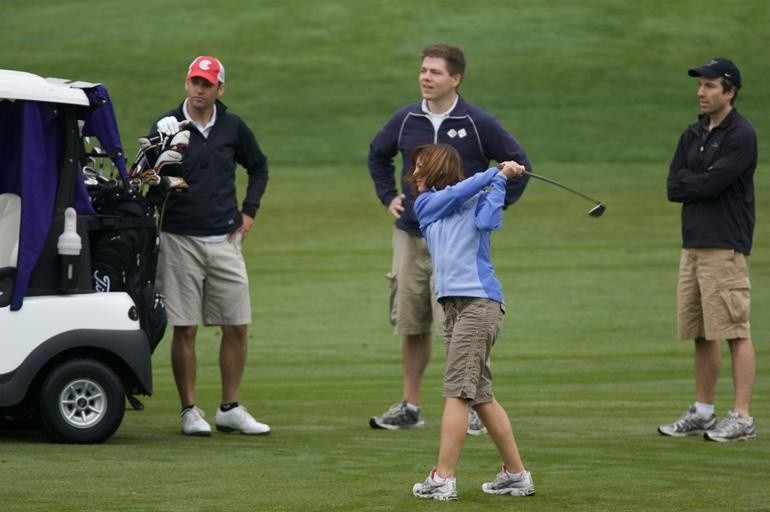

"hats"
[186,56,225,85]
[688,58,740,79]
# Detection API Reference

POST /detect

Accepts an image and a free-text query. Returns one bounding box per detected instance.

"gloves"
[157,116,190,136]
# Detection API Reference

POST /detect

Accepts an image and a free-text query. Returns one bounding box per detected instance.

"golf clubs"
[82,130,190,196]
[498,164,606,217]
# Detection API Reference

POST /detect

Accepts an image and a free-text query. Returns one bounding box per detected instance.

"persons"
[408,143,536,502]
[131,55,271,437]
[657,57,758,444]
[368,43,531,435]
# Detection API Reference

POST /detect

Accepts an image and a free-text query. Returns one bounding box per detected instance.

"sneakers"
[703,410,757,442]
[658,407,717,436]
[466,410,488,436]
[216,405,271,435]
[412,467,459,501]
[482,463,536,497]
[180,406,212,436]
[370,400,425,430]
